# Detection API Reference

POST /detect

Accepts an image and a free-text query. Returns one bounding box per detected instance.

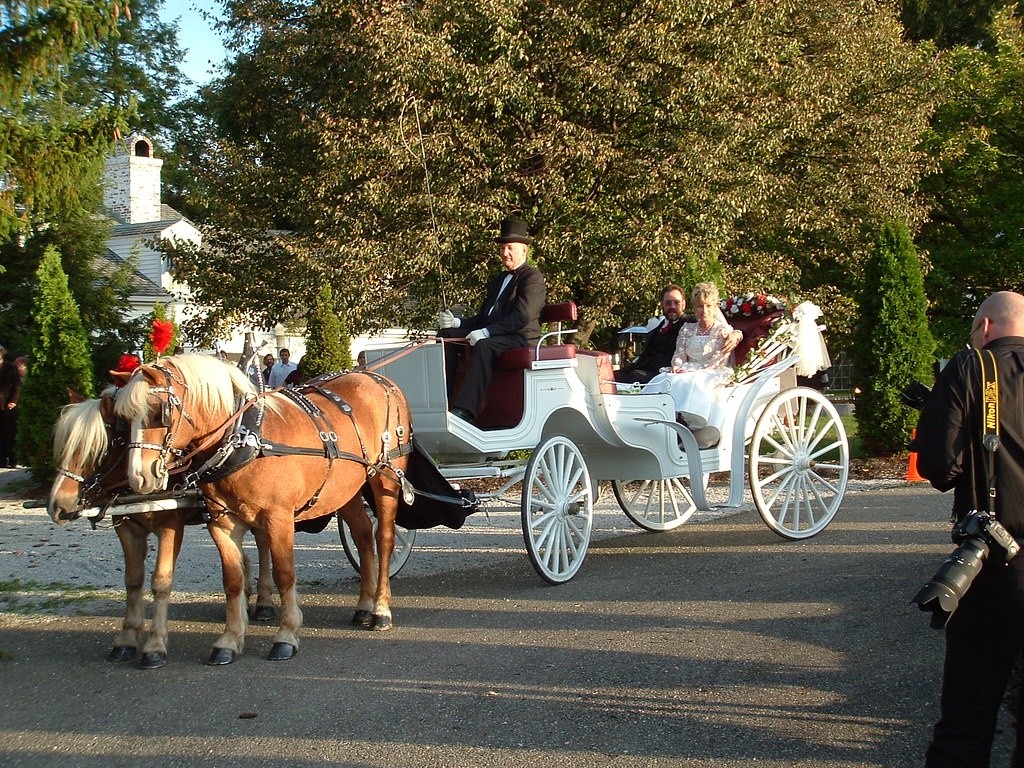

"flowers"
[717,291,797,379]
[111,304,180,389]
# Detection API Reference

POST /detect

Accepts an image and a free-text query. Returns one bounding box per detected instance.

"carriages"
[44,296,851,670]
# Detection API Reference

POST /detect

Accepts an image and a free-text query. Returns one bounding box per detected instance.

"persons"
[352,351,367,372]
[437,219,547,424]
[909,291,1024,768]
[0,344,30,468]
[611,281,744,446]
[259,347,300,388]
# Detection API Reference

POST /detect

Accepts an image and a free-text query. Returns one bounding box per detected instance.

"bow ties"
[505,270,515,276]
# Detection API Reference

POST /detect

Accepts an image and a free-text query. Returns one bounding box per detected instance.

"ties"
[662,321,673,334]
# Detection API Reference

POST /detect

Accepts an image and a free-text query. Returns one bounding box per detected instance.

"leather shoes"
[450,407,475,423]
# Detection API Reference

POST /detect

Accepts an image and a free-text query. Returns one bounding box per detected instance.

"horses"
[46,388,278,668]
[108,353,416,666]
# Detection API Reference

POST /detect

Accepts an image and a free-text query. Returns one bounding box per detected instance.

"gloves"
[465,327,490,345]
[439,309,460,328]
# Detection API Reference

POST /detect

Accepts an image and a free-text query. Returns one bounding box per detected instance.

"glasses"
[663,299,683,306]
[964,318,994,349]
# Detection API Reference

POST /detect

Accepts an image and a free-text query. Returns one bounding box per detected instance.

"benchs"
[728,308,792,380]
[454,300,578,429]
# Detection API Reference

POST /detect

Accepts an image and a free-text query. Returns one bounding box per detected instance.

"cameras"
[908,510,1020,630]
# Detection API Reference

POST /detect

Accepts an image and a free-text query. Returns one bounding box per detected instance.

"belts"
[989,538,1024,546]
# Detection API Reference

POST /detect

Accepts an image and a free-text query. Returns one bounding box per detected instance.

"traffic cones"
[902,428,930,483]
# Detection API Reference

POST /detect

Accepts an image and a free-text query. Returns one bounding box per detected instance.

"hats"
[109,354,140,374]
[492,219,532,244]
